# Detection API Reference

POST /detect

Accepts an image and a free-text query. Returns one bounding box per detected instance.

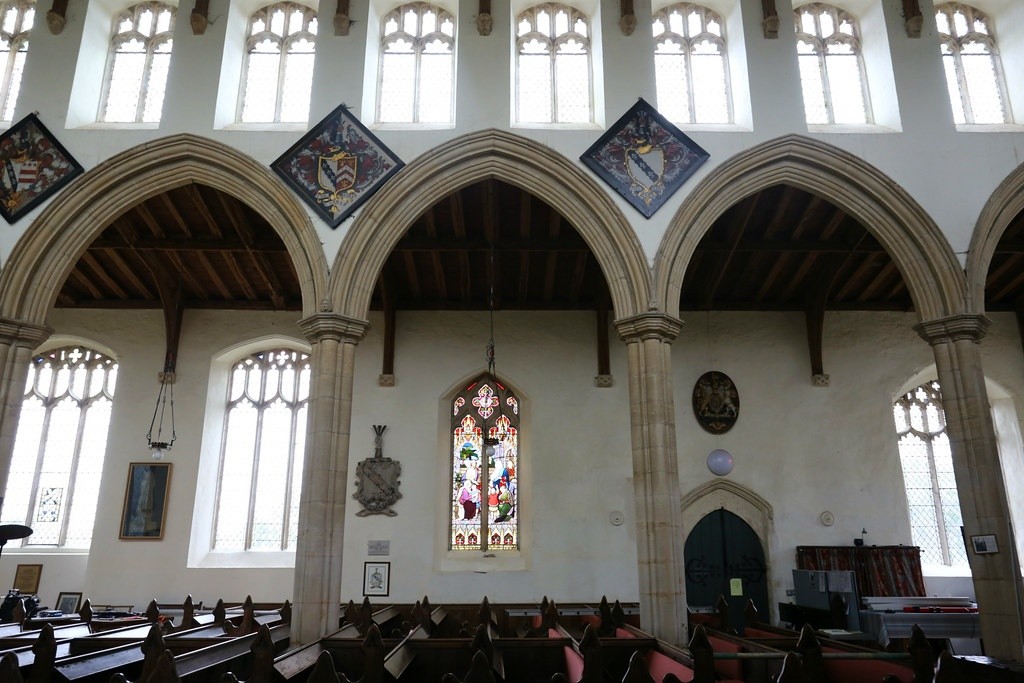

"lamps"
[146,356,177,460]
[706,448,734,477]
[481,253,508,456]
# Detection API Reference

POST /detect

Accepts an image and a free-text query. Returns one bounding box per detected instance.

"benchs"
[0,595,1024,683]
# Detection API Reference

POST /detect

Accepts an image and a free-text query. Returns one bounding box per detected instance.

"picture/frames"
[54,591,83,614]
[971,534,1000,555]
[268,105,406,227]
[363,562,390,597]
[0,112,84,224]
[577,94,710,221]
[119,463,173,541]
[12,563,43,595]
